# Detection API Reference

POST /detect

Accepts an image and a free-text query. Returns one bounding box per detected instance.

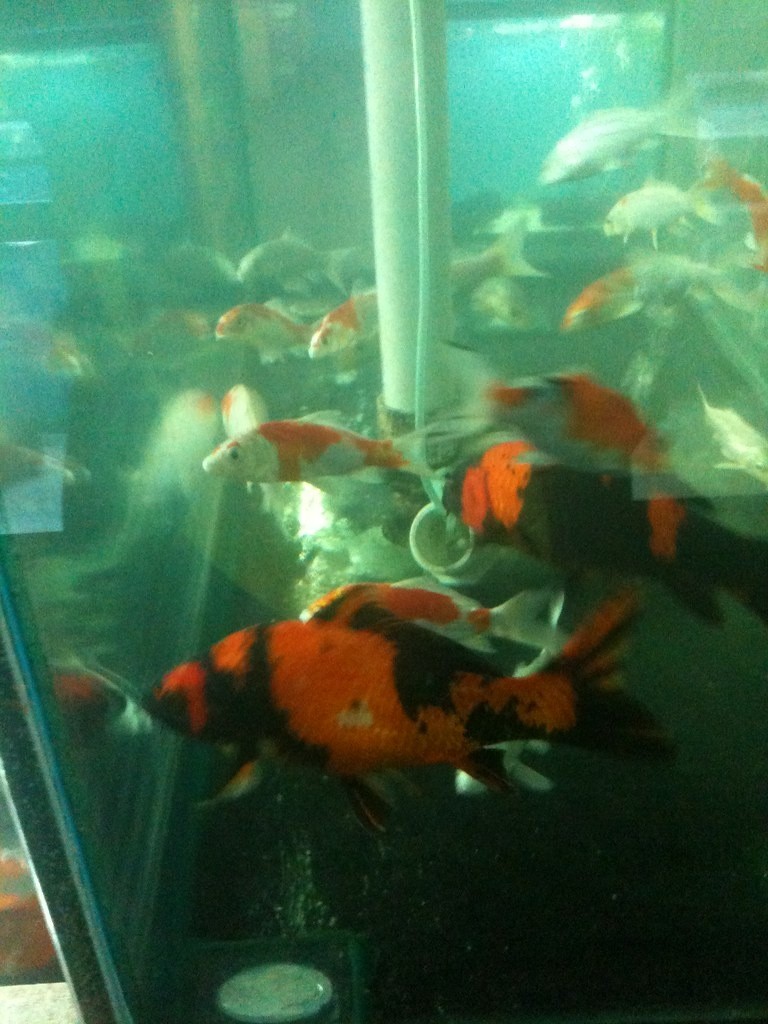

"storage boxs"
[0,0,768,1024]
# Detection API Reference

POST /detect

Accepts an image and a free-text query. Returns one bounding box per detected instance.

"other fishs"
[2,83,766,973]
